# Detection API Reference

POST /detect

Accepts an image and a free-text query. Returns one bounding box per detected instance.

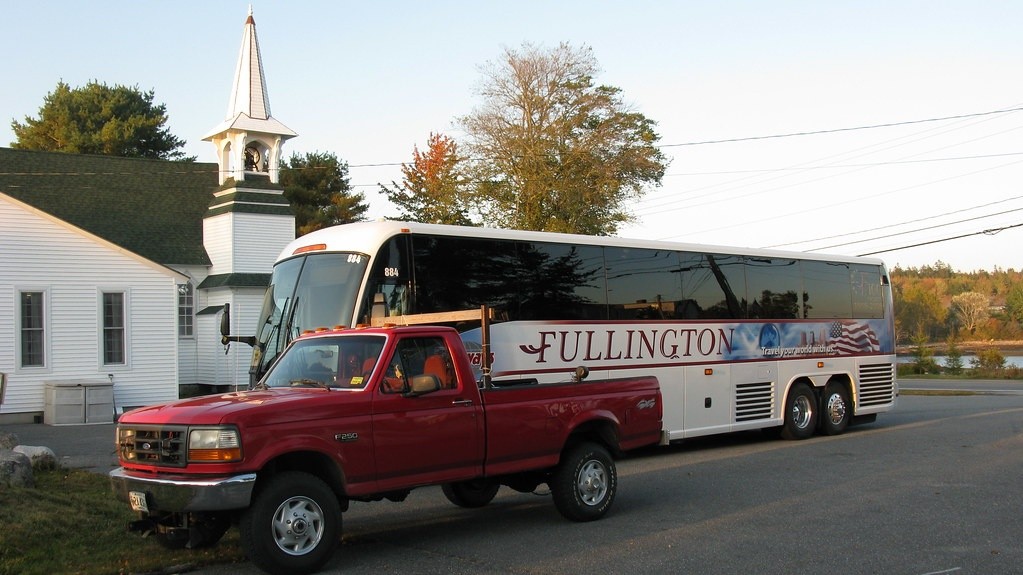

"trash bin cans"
[43,377,115,427]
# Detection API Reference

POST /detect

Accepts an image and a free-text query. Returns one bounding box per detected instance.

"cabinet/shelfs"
[43,377,114,427]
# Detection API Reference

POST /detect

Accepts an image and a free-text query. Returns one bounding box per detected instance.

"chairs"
[421,356,452,389]
[364,356,387,387]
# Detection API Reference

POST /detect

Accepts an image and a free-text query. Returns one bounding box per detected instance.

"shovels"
[108,374,124,422]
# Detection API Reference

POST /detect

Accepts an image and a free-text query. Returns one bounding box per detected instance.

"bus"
[220,216,898,442]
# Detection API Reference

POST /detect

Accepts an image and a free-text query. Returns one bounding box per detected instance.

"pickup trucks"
[108,304,665,575]
